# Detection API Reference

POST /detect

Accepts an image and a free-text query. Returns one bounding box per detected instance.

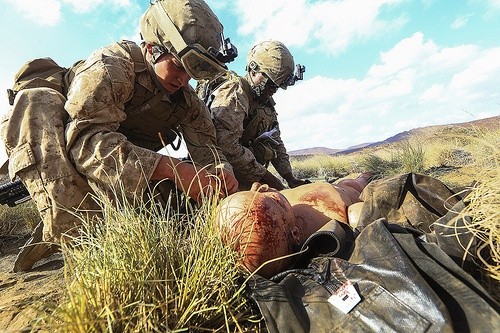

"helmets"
[140,0,223,67]
[247,41,295,90]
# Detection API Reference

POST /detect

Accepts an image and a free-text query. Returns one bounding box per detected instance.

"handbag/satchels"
[7,56,70,105]
[248,141,277,163]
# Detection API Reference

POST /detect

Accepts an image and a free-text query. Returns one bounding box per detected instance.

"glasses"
[176,42,228,83]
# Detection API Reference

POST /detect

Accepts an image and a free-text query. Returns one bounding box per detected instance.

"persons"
[197,40,309,188]
[0,0,238,253]
[214,173,370,275]
